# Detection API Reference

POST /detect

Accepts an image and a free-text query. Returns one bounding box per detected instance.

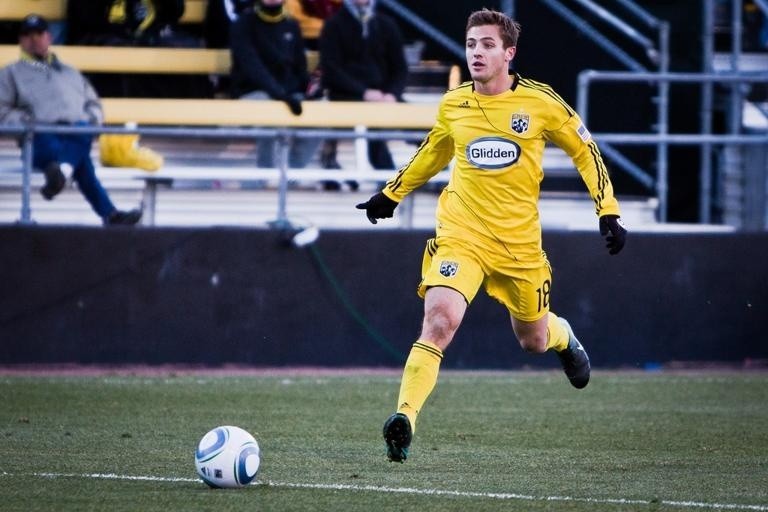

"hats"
[22,14,45,35]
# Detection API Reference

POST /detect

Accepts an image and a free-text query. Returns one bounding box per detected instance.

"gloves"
[601,214,626,256]
[356,193,396,224]
[268,83,301,114]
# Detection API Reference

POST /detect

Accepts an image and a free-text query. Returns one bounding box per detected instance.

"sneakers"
[557,318,591,389]
[383,412,411,463]
[105,210,142,224]
[41,180,64,199]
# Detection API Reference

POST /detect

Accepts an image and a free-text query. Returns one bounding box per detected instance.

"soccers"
[196,425,261,486]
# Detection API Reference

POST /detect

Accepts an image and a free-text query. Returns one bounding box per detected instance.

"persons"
[354,8,628,465]
[0,12,142,226]
[65,1,410,193]
